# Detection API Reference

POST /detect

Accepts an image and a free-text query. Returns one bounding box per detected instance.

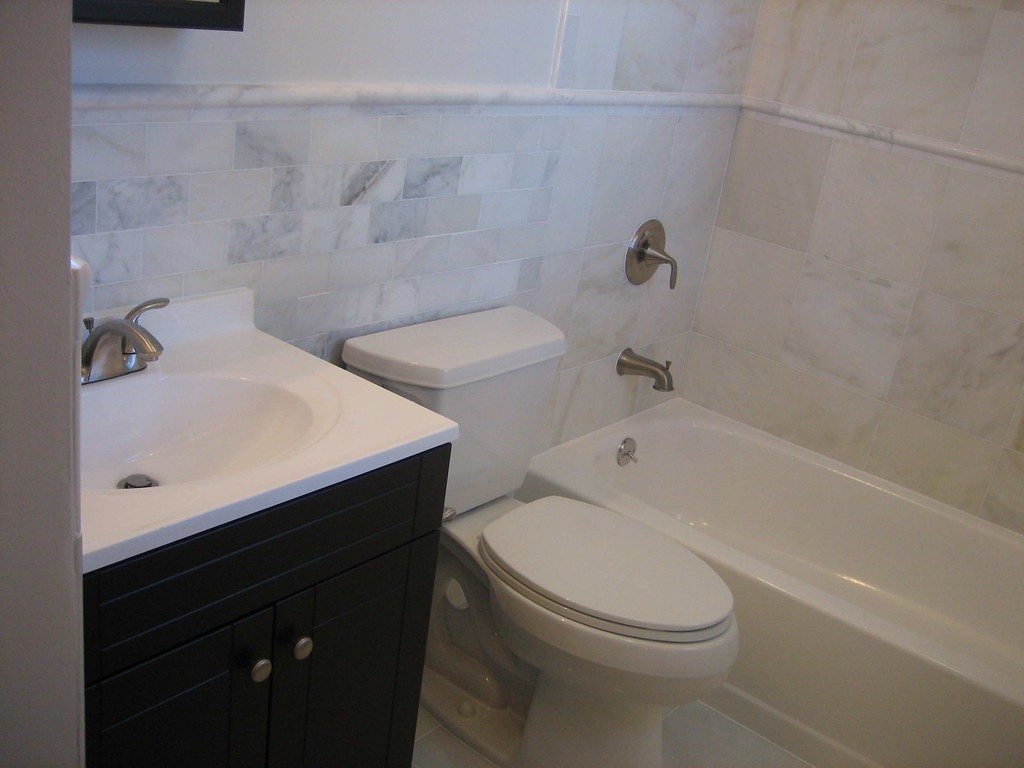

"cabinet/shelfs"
[81,439,454,767]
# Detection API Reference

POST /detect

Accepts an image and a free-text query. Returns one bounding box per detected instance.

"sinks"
[81,379,313,489]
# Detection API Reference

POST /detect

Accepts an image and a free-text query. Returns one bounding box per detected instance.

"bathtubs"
[531,398,1022,762]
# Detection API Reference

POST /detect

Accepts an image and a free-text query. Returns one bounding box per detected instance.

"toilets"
[340,303,738,757]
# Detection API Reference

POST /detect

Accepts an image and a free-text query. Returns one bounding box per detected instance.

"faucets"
[83,322,161,363]
[617,348,677,392]
